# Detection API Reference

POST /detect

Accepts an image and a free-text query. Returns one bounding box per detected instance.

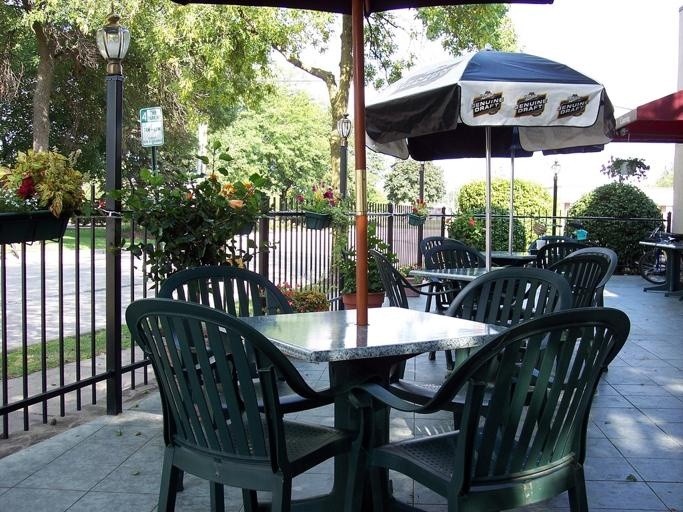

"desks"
[218,303,508,512]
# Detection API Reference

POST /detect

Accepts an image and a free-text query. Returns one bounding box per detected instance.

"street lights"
[336,113,352,311]
[550,156,561,240]
[417,158,425,267]
[94,8,135,414]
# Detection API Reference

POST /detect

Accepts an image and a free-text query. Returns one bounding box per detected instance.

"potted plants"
[338,239,393,308]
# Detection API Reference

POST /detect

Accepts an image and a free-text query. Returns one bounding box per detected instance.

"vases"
[407,213,426,227]
[301,209,325,228]
[0,209,69,244]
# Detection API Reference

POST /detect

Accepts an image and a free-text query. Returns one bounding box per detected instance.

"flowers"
[295,184,338,216]
[0,144,89,222]
[408,197,428,213]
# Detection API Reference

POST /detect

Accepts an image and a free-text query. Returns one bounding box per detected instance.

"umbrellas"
[172,0,554,327]
[612,89,681,145]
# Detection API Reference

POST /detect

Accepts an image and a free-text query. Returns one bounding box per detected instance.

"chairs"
[153,262,293,424]
[389,266,571,455]
[123,296,357,512]
[348,302,633,511]
[368,235,618,373]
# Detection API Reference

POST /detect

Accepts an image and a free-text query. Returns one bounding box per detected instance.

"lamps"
[95,0,131,78]
[336,113,352,149]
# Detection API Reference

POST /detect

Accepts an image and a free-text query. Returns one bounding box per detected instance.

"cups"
[536,240,545,249]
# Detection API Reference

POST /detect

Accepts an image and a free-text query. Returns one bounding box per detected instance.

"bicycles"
[636,229,669,285]
